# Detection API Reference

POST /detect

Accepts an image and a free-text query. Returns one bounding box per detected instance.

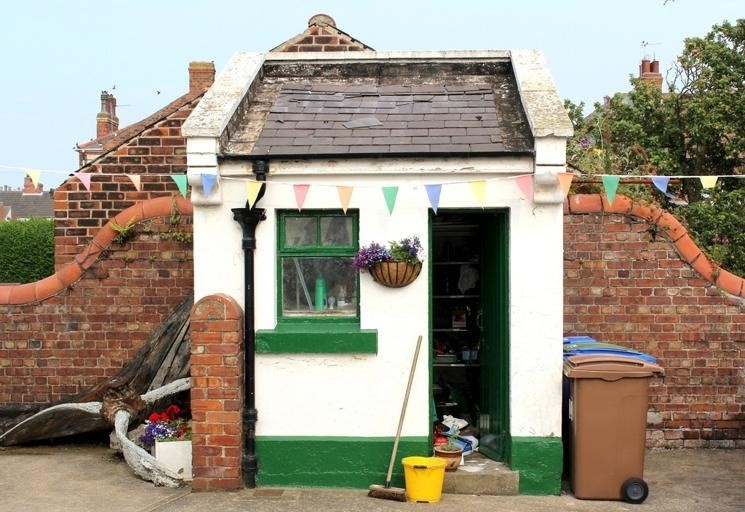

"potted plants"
[434,422,463,473]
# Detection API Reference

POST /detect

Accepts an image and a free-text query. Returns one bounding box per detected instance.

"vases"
[155,439,195,484]
[368,260,422,288]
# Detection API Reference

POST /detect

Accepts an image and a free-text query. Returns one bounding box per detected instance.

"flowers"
[351,235,424,273]
[140,404,190,447]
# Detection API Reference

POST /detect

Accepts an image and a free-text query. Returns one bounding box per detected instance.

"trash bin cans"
[565,355,665,505]
[565,335,658,365]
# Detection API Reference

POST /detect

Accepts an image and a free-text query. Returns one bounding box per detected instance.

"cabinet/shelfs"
[433,223,480,410]
